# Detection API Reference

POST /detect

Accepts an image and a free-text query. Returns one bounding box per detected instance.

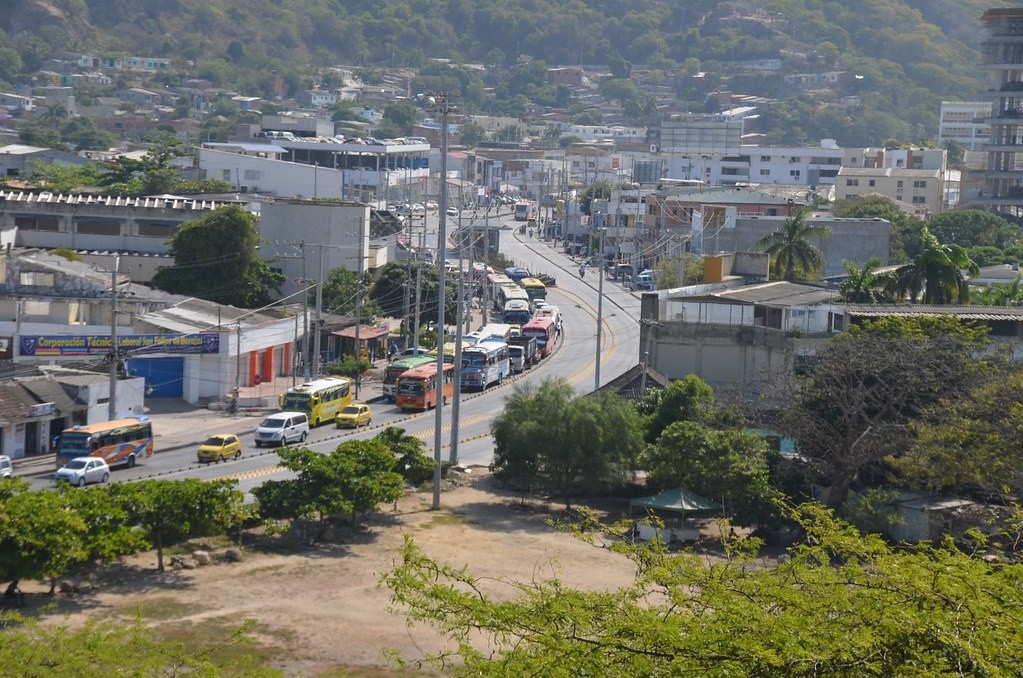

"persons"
[604,264,609,276]
[578,266,585,274]
[358,374,361,392]
[389,341,397,361]
[496,208,500,220]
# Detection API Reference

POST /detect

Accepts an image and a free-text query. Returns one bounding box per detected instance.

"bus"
[543,193,559,207]
[395,362,455,411]
[279,375,353,428]
[53,414,154,467]
[381,353,454,401]
[514,200,538,220]
[427,259,562,391]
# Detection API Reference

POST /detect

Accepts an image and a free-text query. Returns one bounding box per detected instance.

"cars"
[504,267,529,284]
[446,206,458,216]
[0,455,13,480]
[638,270,660,280]
[255,412,309,447]
[624,275,653,288]
[609,264,634,276]
[335,404,371,428]
[390,348,429,363]
[198,433,242,464]
[54,457,110,487]
[533,298,547,309]
[387,199,438,213]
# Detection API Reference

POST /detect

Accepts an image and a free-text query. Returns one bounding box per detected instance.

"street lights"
[424,91,463,510]
